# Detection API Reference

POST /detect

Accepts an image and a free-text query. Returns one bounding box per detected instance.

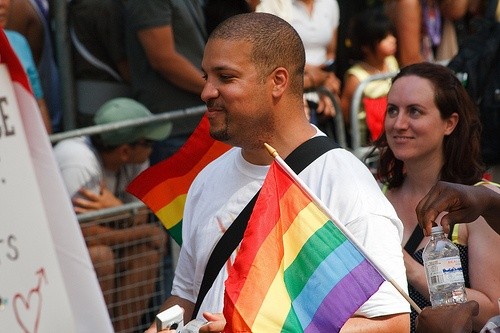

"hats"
[94,98,173,144]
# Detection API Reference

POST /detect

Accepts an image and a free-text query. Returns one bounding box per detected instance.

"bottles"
[422,226,467,308]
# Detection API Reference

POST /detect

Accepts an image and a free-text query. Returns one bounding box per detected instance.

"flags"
[223,142,421,333]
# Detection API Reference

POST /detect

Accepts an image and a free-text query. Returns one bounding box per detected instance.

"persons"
[67,0,137,91]
[365,62,500,333]
[53,98,173,333]
[338,13,402,143]
[145,13,411,332]
[414,181,500,333]
[122,0,210,167]
[386,0,500,168]
[256,0,340,127]
[0,29,55,135]
[0,0,63,125]
[205,0,260,35]
